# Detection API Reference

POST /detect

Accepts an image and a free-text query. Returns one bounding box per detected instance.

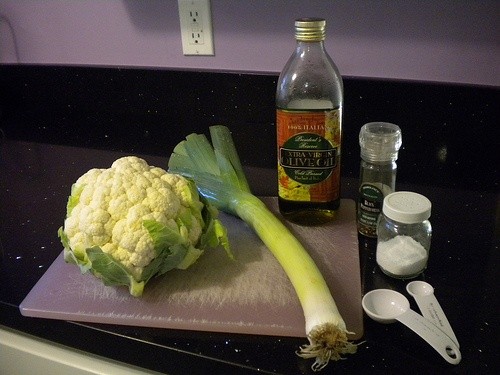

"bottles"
[374,192,435,280]
[277,17,341,229]
[361,123,403,236]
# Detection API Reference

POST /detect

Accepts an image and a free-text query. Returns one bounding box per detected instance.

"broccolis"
[57,155,234,297]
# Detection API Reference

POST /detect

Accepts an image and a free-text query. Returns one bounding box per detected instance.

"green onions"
[167,124,368,372]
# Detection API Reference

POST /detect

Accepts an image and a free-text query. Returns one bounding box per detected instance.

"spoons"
[406,281,461,355]
[362,288,462,365]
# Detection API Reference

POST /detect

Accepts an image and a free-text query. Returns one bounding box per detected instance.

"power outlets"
[177,0,215,56]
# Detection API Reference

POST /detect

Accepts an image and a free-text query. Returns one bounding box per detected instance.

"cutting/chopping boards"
[17,187,362,342]
